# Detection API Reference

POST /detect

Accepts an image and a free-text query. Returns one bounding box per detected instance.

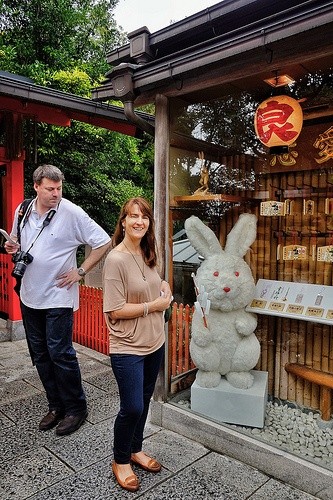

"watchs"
[78,268,86,276]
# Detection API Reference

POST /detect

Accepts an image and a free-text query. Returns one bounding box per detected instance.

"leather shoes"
[56,412,88,435]
[39,410,65,431]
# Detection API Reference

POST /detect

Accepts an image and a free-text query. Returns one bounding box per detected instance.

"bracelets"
[142,302,149,317]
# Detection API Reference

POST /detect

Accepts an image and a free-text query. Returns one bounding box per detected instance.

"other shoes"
[130,451,162,473]
[112,459,139,491]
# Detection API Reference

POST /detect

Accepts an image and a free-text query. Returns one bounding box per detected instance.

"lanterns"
[254,94,303,155]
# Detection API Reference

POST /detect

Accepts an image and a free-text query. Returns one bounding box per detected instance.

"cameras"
[11,251,33,279]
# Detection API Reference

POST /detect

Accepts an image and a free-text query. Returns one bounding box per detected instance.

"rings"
[69,279,71,281]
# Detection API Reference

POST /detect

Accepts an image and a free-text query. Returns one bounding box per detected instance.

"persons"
[103,198,172,492]
[3,165,112,434]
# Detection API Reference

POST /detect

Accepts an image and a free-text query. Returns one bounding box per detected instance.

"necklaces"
[123,242,146,281]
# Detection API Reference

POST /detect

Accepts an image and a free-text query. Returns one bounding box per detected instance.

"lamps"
[265,74,295,86]
